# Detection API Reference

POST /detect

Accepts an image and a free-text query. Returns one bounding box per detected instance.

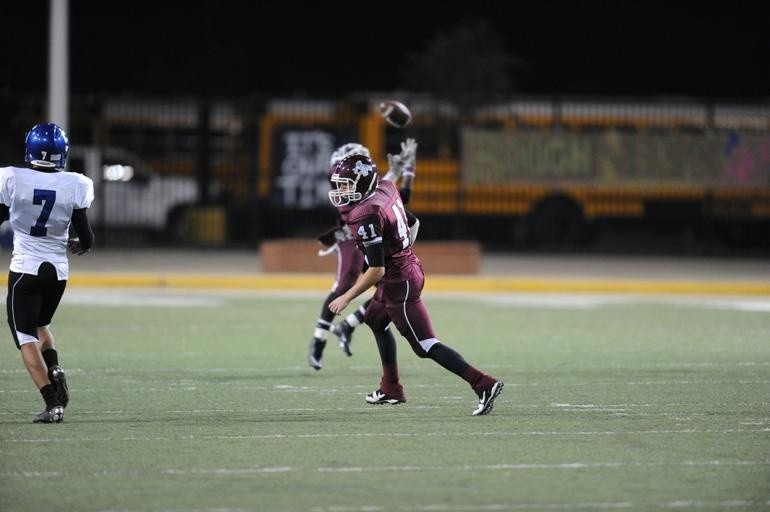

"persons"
[305,137,418,371]
[0,120,97,425]
[328,153,505,415]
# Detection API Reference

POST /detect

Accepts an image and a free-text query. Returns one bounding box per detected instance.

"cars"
[68,145,201,240]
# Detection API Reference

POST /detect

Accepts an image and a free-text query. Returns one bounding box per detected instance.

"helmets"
[328,142,379,198]
[24,123,69,168]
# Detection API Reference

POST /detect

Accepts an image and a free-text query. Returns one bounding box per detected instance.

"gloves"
[387,138,418,178]
[68,238,89,256]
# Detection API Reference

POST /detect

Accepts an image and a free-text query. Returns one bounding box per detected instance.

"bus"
[253,99,769,253]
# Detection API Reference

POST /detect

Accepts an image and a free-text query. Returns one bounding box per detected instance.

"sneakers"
[472,380,504,416]
[329,320,355,357]
[32,405,64,423]
[309,336,326,370]
[49,364,69,408]
[366,389,406,405]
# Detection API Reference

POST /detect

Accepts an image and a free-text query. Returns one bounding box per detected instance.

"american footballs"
[380,100,412,129]
